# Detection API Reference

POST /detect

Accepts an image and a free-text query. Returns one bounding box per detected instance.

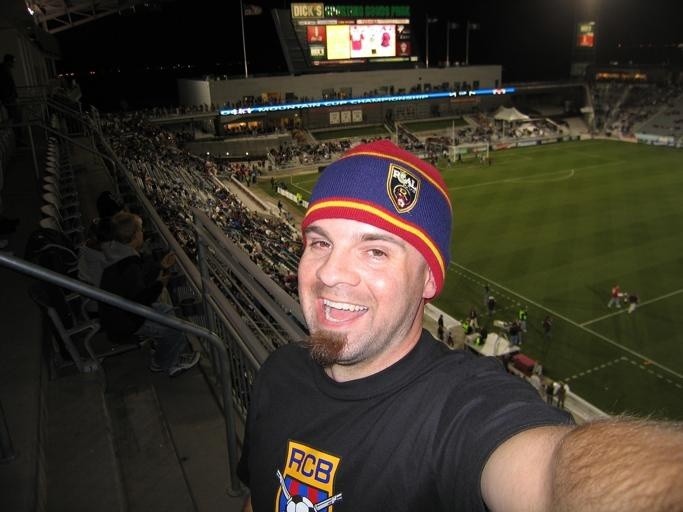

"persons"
[234,140,682,510]
[54,83,479,128]
[98,214,202,376]
[436,281,567,412]
[268,119,564,170]
[607,283,622,310]
[349,27,364,58]
[379,26,391,56]
[627,292,639,313]
[131,180,307,326]
[105,128,262,179]
[73,191,126,313]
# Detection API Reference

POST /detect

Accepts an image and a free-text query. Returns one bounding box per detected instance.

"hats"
[301,139,451,303]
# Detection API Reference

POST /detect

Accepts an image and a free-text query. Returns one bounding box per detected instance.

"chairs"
[24,114,304,380]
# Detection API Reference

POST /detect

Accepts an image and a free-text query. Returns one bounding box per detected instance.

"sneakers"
[151,349,202,377]
